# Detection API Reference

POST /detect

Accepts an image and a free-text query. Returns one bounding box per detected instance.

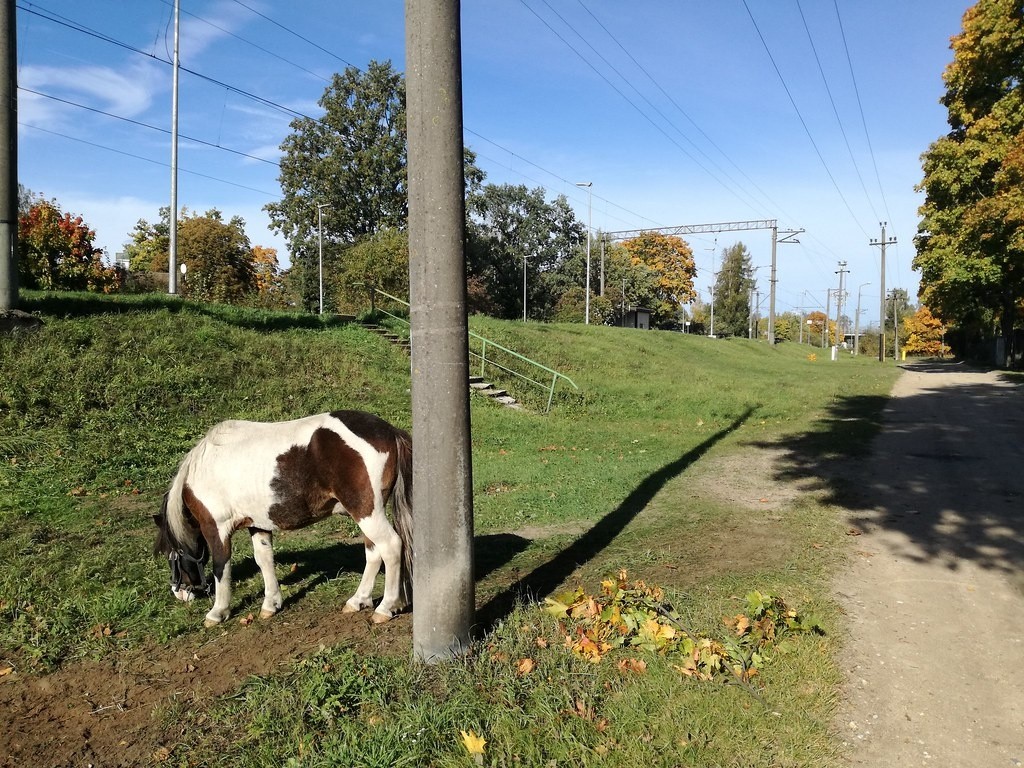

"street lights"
[576,182,593,325]
[852,281,872,355]
[704,248,716,338]
[621,279,630,328]
[523,254,532,322]
[318,203,331,314]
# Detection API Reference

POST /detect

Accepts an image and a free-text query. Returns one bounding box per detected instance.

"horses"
[152,410,415,628]
